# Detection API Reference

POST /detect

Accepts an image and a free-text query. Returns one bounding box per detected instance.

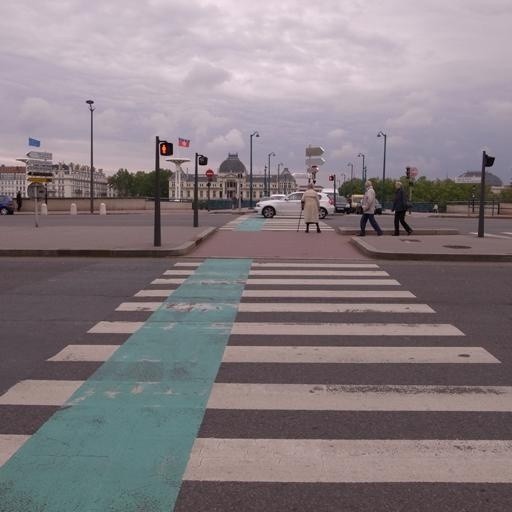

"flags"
[179,138,191,147]
[29,138,40,148]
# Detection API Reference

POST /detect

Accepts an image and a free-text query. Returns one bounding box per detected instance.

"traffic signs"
[25,151,53,184]
[305,146,325,166]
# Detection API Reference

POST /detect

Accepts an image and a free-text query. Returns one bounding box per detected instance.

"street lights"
[249,130,260,207]
[267,151,298,197]
[337,161,354,196]
[357,153,365,193]
[377,131,386,211]
[86,100,96,212]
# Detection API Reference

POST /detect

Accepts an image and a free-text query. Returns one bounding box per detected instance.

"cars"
[0,195,15,215]
[254,188,382,219]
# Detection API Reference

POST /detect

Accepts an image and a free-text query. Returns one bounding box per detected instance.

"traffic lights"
[405,167,410,179]
[329,176,334,181]
[160,142,173,156]
[199,157,207,166]
[484,156,495,167]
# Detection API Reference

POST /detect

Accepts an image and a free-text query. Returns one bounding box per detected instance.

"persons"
[356,181,384,236]
[434,204,438,213]
[301,183,321,233]
[391,181,413,236]
[16,191,22,211]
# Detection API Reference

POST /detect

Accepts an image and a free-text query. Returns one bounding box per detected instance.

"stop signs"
[411,167,418,177]
[206,170,214,177]
